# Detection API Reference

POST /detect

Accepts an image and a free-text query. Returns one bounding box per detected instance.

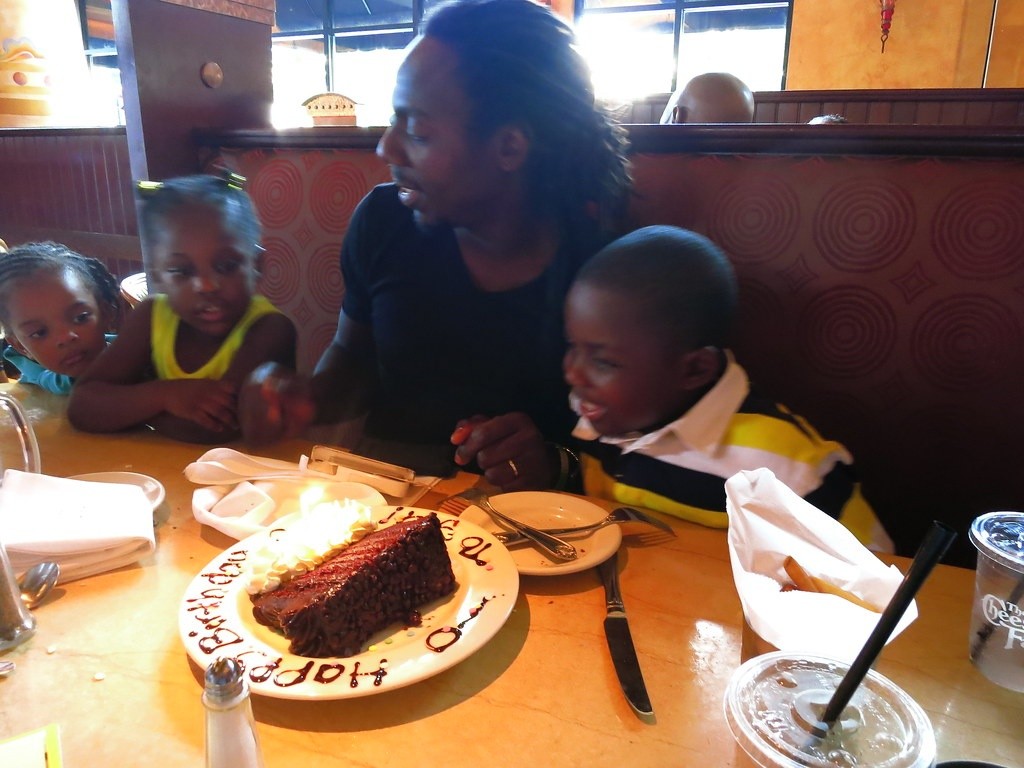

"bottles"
[201,659,262,768]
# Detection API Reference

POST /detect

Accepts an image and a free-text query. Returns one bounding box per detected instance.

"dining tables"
[0,383,1024,768]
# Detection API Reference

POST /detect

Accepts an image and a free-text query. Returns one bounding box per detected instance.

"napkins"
[0,468,157,587]
[181,447,388,542]
[722,468,920,667]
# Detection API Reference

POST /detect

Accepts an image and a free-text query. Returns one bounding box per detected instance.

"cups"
[0,392,41,490]
[0,542,38,655]
[969,512,1024,693]
[723,650,937,768]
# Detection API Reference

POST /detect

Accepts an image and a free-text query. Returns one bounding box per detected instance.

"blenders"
[408,478,441,507]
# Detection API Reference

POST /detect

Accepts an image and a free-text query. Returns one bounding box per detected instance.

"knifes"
[596,551,653,715]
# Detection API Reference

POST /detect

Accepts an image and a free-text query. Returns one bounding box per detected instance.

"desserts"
[245,499,457,656]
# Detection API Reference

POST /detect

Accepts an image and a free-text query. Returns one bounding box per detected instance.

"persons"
[237,1,643,490]
[659,73,758,125]
[559,223,899,555]
[1,240,135,397]
[66,174,300,446]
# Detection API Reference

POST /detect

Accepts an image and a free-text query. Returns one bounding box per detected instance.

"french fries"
[777,556,881,613]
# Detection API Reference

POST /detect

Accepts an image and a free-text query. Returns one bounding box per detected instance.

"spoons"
[18,560,60,608]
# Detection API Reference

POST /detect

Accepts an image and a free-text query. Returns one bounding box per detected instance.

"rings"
[507,458,520,478]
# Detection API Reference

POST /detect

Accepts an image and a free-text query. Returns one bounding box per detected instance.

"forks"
[438,488,577,560]
[494,508,678,548]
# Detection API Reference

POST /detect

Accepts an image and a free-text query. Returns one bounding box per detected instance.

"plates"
[66,472,166,512]
[352,482,388,507]
[178,506,519,701]
[488,491,622,575]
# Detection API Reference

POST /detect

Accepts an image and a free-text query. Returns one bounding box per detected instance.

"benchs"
[209,126,1024,569]
